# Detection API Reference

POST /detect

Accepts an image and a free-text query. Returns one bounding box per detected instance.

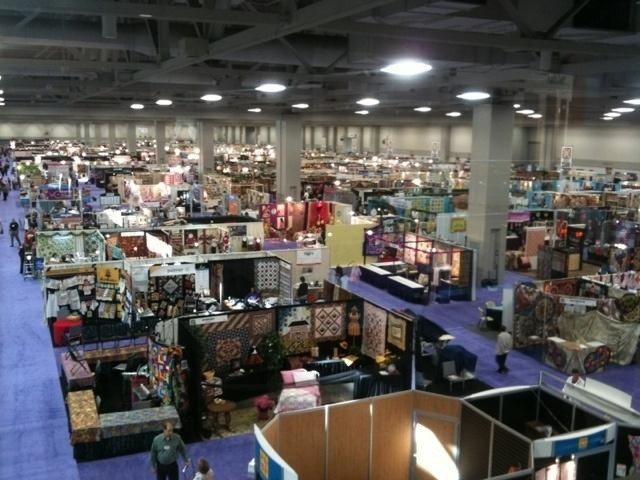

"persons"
[562,368,585,401]
[298,275,310,304]
[18,244,24,274]
[9,218,21,247]
[191,456,215,480]
[0,143,13,201]
[495,325,514,373]
[149,420,192,480]
[246,287,259,298]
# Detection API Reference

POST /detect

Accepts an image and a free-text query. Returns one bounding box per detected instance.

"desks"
[7,137,640,480]
